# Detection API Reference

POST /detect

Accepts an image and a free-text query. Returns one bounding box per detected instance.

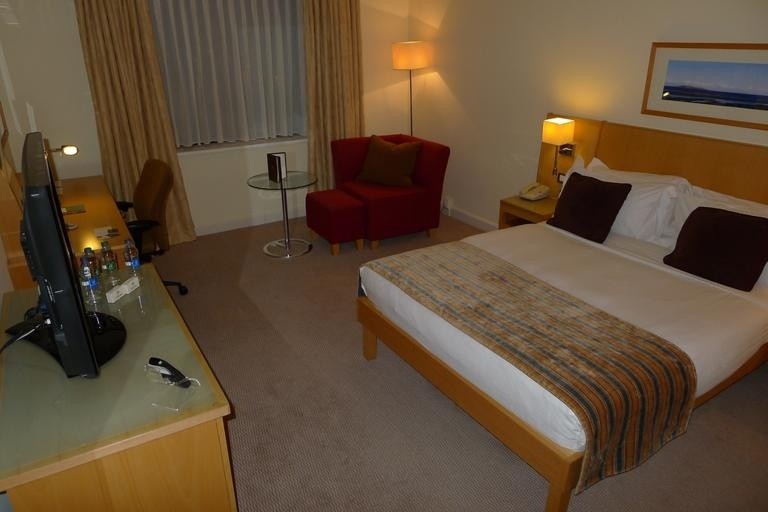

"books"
[61,204,86,215]
[267,152,289,182]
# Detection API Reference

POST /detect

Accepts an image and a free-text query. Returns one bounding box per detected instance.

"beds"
[357,112,768,512]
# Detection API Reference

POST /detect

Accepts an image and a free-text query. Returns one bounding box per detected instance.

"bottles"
[122,240,145,285]
[80,247,103,313]
[99,241,121,286]
[133,294,152,325]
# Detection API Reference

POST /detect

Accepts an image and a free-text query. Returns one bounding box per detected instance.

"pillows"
[353,134,425,187]
[547,155,768,292]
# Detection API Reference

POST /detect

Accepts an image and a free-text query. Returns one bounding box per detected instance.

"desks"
[246,172,319,260]
[1,149,138,290]
[1,262,240,512]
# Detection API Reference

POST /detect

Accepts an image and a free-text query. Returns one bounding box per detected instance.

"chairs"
[115,159,189,296]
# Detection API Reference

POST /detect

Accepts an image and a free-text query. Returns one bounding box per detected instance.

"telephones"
[520,183,550,201]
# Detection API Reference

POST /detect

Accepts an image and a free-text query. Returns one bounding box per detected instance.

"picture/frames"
[639,40,768,131]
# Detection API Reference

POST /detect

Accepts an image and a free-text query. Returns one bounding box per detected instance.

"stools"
[305,189,365,256]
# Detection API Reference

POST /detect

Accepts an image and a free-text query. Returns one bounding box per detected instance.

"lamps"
[390,40,434,135]
[541,116,575,177]
[46,144,82,159]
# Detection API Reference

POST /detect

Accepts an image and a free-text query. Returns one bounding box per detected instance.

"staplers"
[149,357,190,388]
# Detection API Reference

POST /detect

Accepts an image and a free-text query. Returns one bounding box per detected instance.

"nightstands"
[497,195,557,231]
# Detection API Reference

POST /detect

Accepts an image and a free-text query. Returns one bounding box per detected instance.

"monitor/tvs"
[5,131,127,379]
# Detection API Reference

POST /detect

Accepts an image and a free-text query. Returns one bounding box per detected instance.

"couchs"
[331,133,450,251]
